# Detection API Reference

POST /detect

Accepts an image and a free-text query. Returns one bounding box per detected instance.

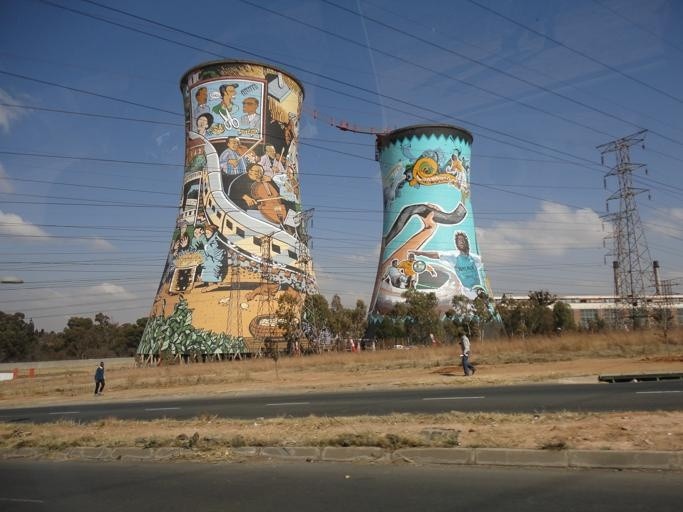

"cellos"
[251,168,287,223]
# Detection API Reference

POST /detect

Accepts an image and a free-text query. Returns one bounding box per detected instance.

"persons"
[349,336,357,352]
[93,361,106,396]
[160,82,302,294]
[457,328,477,377]
[397,253,437,289]
[360,336,366,352]
[446,153,468,190]
[408,231,485,310]
[292,337,303,360]
[381,259,405,287]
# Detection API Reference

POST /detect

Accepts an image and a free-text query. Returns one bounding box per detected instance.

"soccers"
[413,260,427,273]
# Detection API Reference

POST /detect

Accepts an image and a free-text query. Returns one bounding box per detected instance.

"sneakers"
[94,392,104,396]
[464,367,477,377]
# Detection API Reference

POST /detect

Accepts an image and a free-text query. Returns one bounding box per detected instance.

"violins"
[291,179,299,199]
[238,145,260,162]
[276,153,288,164]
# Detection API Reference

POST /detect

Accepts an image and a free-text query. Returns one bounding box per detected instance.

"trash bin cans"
[361,339,374,353]
